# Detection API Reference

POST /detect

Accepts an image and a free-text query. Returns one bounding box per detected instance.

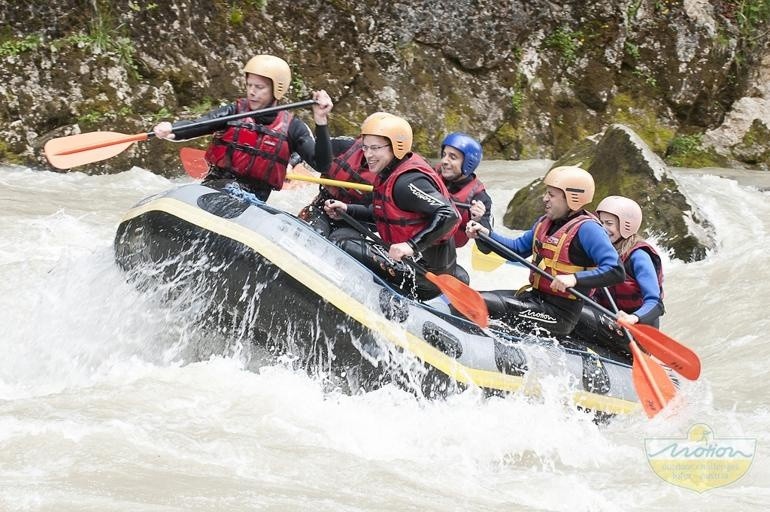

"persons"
[324,111,462,301]
[284,135,379,246]
[433,133,492,286]
[571,196,667,363]
[153,54,333,204]
[448,166,626,340]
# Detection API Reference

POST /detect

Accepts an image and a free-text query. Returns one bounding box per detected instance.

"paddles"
[472,222,701,381]
[330,198,488,329]
[44,100,315,170]
[179,147,373,192]
[602,287,677,419]
[471,245,530,271]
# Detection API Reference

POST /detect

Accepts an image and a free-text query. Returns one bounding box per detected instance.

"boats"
[113,177,681,429]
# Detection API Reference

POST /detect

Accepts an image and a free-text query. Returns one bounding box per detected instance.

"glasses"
[360,142,392,151]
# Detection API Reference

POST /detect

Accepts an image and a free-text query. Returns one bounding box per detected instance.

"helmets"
[440,133,483,177]
[359,111,414,160]
[597,195,644,239]
[243,53,293,100]
[542,164,596,210]
